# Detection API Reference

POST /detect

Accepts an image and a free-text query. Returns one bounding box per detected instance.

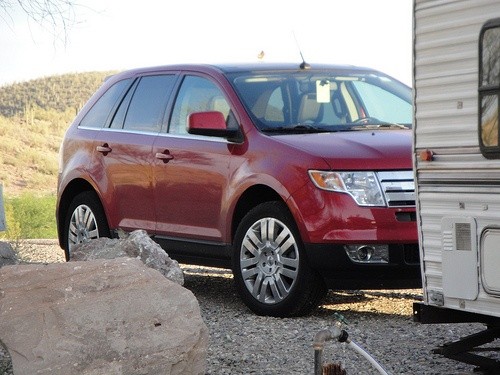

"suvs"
[51,59,423,318]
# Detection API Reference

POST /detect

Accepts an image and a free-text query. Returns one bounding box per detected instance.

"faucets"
[313,311,349,375]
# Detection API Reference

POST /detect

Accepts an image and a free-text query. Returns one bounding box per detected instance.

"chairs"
[206,95,231,124]
[293,92,324,126]
